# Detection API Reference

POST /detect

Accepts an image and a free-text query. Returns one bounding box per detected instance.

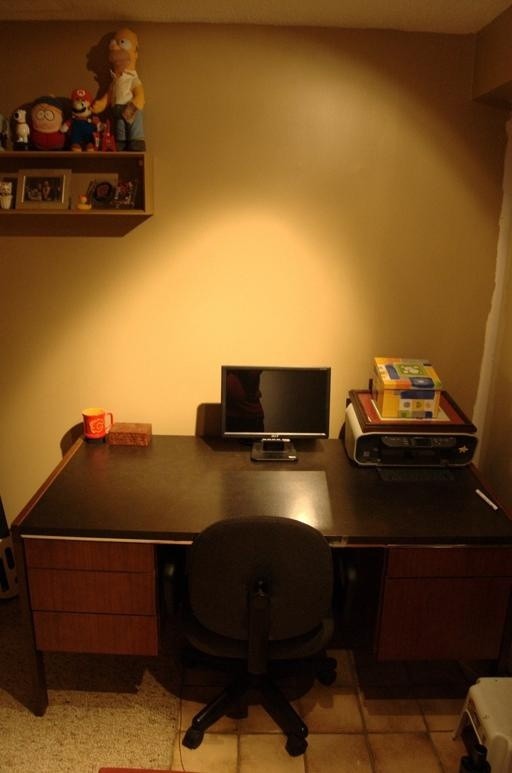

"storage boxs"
[370,357,442,418]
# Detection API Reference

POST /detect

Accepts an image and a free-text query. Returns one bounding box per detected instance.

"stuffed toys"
[0,182,14,210]
[76,194,92,211]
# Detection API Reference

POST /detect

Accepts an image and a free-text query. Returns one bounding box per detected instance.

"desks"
[9,434,509,716]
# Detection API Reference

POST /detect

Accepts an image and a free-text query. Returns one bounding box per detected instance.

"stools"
[453,676,511,773]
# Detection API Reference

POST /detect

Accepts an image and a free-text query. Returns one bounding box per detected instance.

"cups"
[81,406,115,441]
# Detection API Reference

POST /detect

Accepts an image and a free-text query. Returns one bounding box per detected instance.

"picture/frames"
[15,168,72,210]
[348,389,477,435]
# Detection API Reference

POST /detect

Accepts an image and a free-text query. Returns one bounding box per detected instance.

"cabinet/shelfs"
[1,150,154,238]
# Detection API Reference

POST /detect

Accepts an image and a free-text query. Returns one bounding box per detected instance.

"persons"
[0,25,147,152]
[31,179,60,202]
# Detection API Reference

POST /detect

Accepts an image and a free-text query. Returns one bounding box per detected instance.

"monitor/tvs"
[219,365,331,462]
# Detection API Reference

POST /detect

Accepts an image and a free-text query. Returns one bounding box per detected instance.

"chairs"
[162,514,337,756]
